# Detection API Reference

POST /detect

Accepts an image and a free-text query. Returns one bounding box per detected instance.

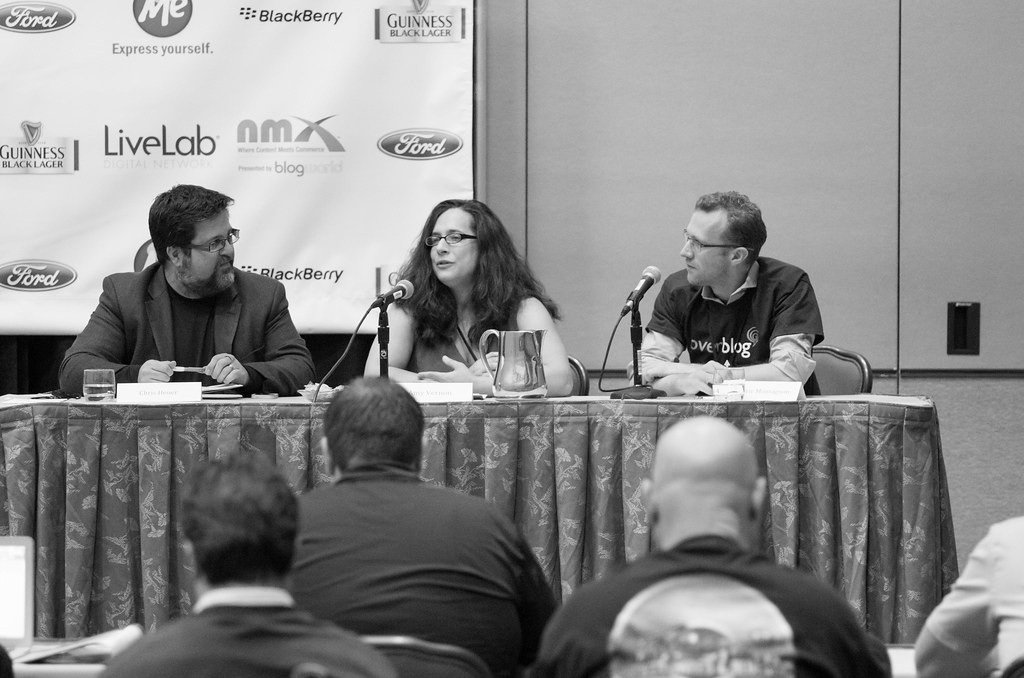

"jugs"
[479,329,547,399]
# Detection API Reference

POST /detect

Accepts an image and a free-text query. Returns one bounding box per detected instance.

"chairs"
[804,346,873,398]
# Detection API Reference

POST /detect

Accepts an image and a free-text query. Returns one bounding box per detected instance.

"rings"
[230,365,235,369]
[226,355,234,360]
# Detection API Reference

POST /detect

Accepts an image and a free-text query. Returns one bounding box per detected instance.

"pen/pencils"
[172,366,205,374]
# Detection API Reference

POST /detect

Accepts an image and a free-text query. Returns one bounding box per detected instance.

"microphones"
[621,266,661,316]
[372,280,415,308]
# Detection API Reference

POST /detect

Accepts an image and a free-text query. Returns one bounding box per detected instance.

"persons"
[58,184,314,396]
[365,198,575,396]
[98,453,398,678]
[537,414,892,678]
[914,515,1024,678]
[291,374,556,678]
[624,190,825,394]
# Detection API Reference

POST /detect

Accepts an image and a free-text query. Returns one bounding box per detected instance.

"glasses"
[683,228,749,253]
[172,228,240,253]
[425,233,477,247]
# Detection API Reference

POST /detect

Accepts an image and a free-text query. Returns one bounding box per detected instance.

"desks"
[0,389,960,643]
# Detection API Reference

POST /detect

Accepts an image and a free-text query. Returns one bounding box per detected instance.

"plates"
[297,390,339,402]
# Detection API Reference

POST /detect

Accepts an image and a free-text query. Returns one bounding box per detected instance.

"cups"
[83,370,115,402]
[713,369,745,400]
